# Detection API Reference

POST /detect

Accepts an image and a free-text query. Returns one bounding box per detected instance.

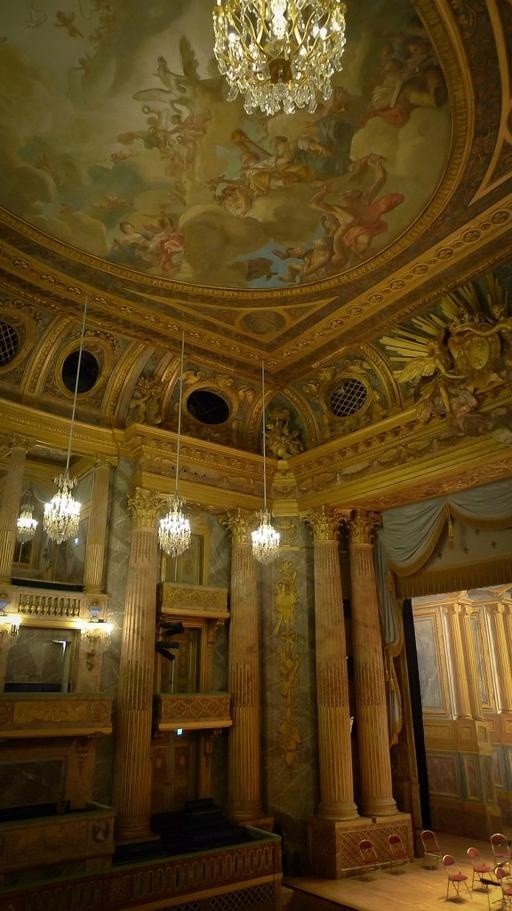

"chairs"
[441,834,512,911]
[357,830,444,881]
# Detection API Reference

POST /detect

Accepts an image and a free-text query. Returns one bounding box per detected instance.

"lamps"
[157,329,192,560]
[42,295,89,545]
[17,496,39,546]
[210,1,348,120]
[250,359,282,569]
